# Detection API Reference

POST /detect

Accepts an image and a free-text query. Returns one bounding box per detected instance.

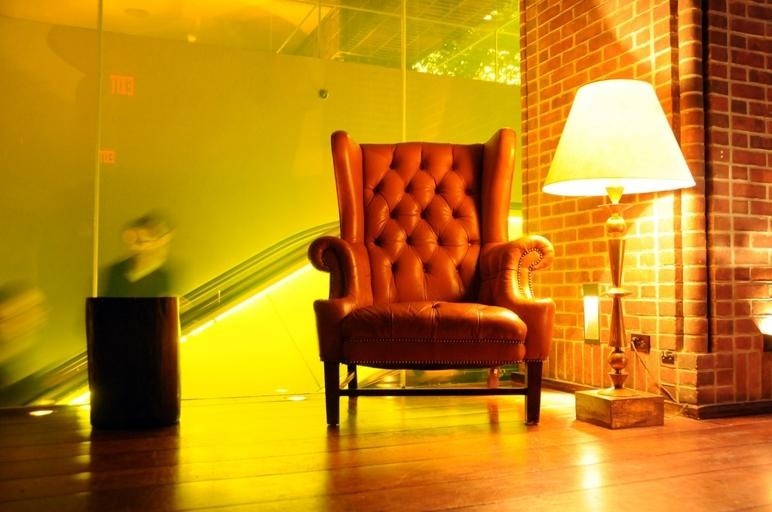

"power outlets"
[629,332,651,354]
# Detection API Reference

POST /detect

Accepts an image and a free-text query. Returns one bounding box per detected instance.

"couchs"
[306,128,557,428]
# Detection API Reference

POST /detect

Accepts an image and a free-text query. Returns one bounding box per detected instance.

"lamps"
[581,283,600,345]
[540,76,699,430]
